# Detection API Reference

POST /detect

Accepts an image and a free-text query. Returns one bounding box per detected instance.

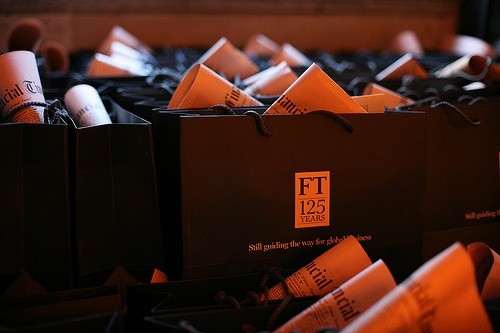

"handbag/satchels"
[0,42,500,333]
